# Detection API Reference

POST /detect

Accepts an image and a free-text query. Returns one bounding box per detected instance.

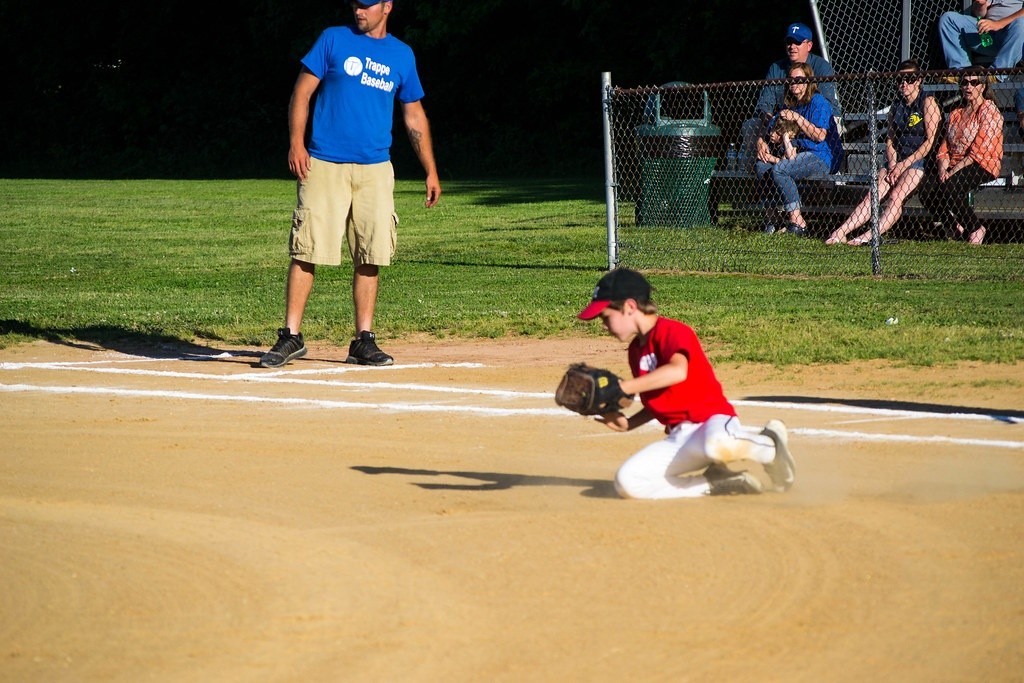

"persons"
[1014,88,1024,137]
[260,0,442,368]
[577,267,795,499]
[919,67,1003,245]
[938,0,1024,83]
[825,60,943,246]
[741,23,844,236]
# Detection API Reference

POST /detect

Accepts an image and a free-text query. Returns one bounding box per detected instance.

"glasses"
[960,78,981,87]
[787,41,807,46]
[896,75,918,84]
[789,76,802,77]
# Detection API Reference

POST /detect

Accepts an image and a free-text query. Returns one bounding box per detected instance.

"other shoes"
[986,75,999,83]
[944,75,961,85]
[968,224,986,245]
[765,224,775,233]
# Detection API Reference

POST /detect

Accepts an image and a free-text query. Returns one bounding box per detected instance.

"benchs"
[703,67,1022,233]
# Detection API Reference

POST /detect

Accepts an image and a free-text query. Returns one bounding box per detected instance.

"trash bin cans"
[631,83,723,227]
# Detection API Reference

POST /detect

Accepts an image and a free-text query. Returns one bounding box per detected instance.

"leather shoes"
[783,222,812,239]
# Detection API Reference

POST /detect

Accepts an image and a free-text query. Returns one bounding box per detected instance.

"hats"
[359,0,389,6]
[576,263,650,319]
[785,23,812,41]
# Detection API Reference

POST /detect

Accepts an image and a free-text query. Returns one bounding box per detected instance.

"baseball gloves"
[555,361,635,416]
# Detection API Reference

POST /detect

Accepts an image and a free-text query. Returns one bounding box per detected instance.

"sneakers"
[703,460,762,494]
[761,416,795,492]
[346,328,394,365]
[259,324,308,369]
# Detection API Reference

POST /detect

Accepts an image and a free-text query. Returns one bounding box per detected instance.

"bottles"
[737,143,750,173]
[726,142,738,172]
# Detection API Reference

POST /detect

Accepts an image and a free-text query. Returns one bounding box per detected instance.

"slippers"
[825,233,848,245]
[845,235,871,246]
